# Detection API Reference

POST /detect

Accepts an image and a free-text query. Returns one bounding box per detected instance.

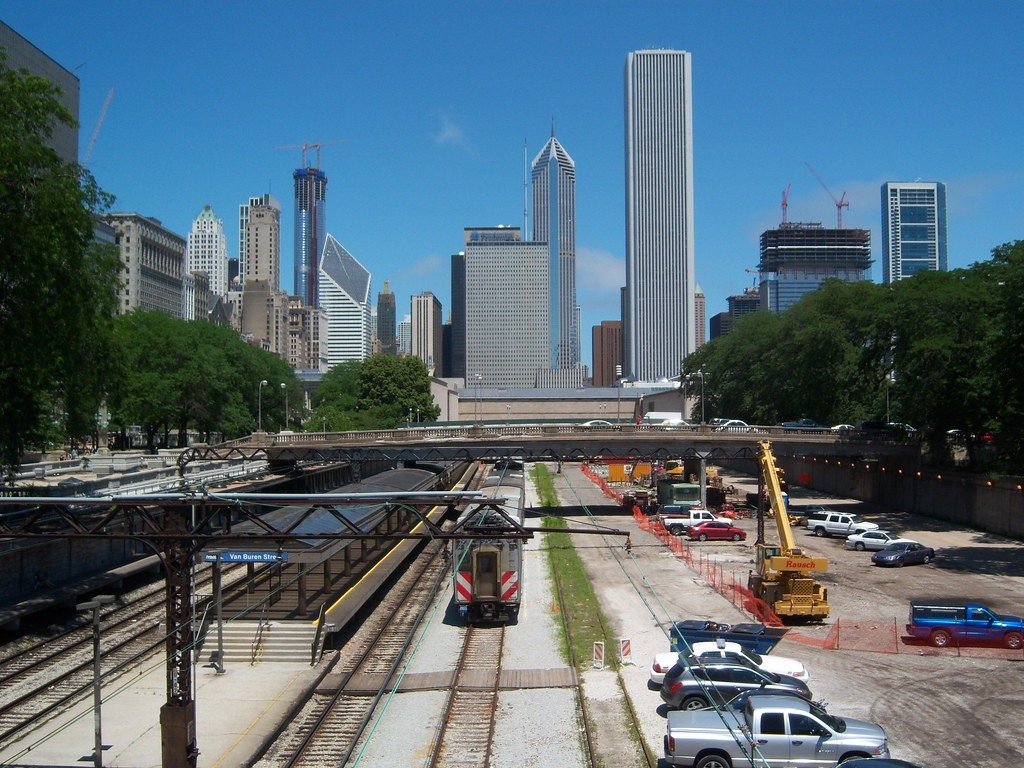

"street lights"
[684,374,691,418]
[477,377,483,424]
[615,363,623,425]
[417,408,420,423]
[474,374,481,424]
[258,380,267,430]
[696,371,705,422]
[884,374,896,424]
[280,382,289,428]
[409,408,412,423]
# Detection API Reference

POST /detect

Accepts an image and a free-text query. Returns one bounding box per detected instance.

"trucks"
[639,411,682,431]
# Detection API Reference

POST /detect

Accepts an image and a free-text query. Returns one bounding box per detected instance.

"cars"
[845,530,918,551]
[686,521,747,542]
[708,417,759,434]
[650,638,810,685]
[871,542,935,569]
[579,419,620,432]
[831,420,993,442]
[661,418,692,431]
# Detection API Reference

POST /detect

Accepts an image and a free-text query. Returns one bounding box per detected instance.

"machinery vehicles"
[747,438,831,626]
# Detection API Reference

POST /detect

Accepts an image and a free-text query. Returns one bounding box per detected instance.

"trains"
[452,457,526,624]
[0,459,394,640]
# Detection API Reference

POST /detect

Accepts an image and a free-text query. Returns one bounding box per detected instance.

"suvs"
[660,656,814,712]
[655,504,706,521]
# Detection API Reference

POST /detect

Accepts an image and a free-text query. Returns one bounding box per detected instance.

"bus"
[656,478,702,506]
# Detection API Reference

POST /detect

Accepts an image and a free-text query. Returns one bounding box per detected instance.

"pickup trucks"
[806,510,880,537]
[782,418,827,434]
[662,694,891,768]
[664,509,734,536]
[905,598,1024,650]
